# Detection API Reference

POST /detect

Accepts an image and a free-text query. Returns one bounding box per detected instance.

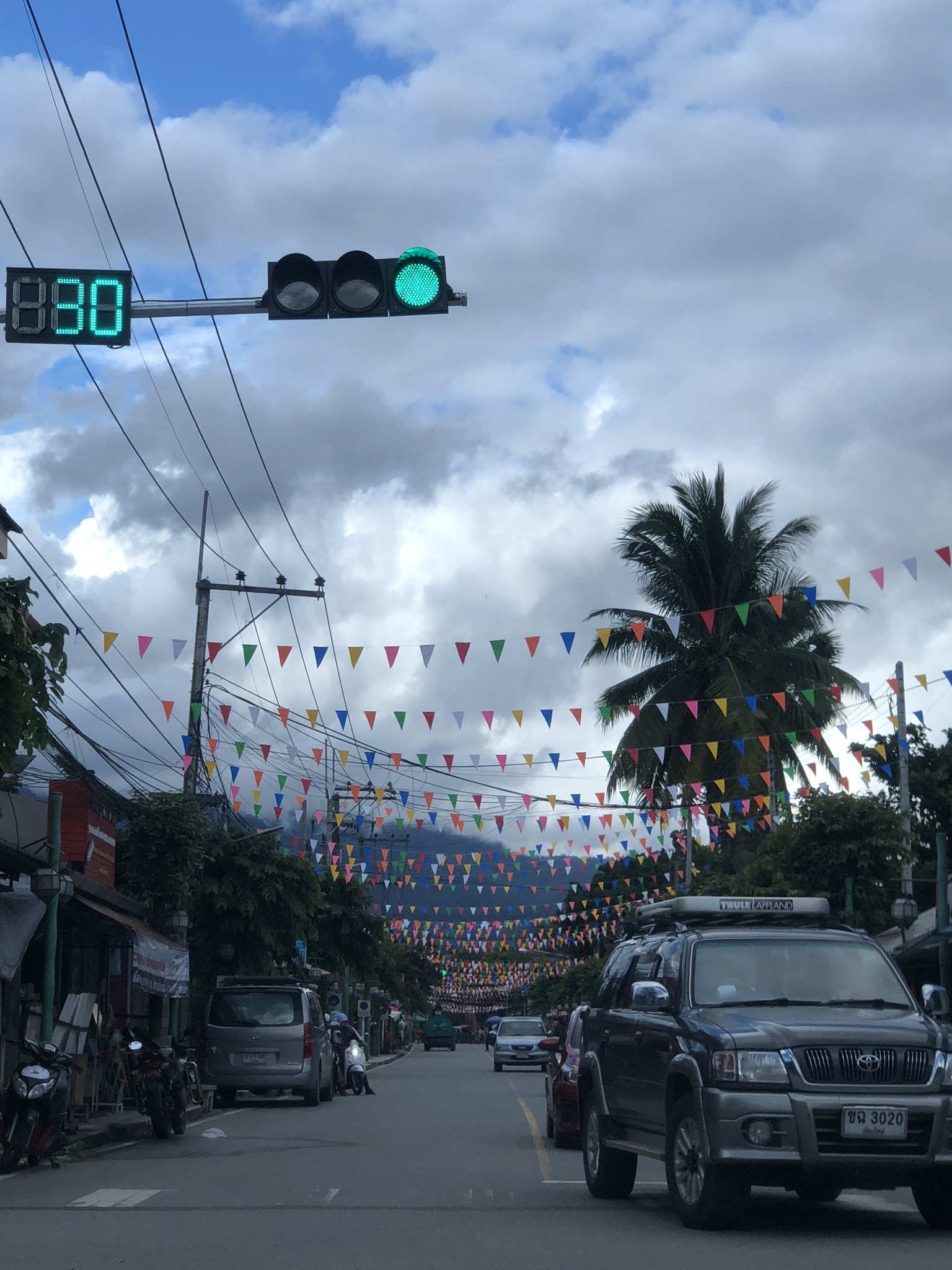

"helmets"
[336,1014,348,1022]
[558,1011,570,1025]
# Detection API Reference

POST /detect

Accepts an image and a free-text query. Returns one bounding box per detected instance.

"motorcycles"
[126,1024,203,1141]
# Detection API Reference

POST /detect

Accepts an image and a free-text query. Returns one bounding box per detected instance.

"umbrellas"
[484,1016,502,1023]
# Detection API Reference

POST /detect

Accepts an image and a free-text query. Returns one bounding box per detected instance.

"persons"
[472,1022,492,1052]
[547,1011,569,1037]
[328,1011,376,1095]
[384,1021,424,1053]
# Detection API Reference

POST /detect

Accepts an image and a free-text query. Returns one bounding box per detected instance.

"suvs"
[488,1017,552,1072]
[578,896,952,1235]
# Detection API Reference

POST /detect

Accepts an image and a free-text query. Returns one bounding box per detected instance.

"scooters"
[335,1031,370,1095]
[1,1037,86,1177]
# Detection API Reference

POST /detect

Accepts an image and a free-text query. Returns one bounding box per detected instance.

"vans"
[202,984,340,1106]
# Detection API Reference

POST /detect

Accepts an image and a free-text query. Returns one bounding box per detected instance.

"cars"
[535,1006,589,1148]
[452,1026,475,1044]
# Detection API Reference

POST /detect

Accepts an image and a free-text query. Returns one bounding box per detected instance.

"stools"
[70,1105,90,1123]
[187,1083,217,1115]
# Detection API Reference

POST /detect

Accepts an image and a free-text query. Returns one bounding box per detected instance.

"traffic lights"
[265,247,449,322]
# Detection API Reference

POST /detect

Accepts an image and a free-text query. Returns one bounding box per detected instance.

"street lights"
[888,831,952,1027]
[330,965,353,1027]
[163,877,189,1044]
[355,982,379,1058]
[184,824,286,1047]
[517,947,575,1013]
[29,788,78,1043]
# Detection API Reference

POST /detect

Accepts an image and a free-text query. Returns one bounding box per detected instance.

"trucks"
[424,1016,456,1051]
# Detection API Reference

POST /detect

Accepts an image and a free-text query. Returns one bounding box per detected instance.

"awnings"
[67,892,190,999]
